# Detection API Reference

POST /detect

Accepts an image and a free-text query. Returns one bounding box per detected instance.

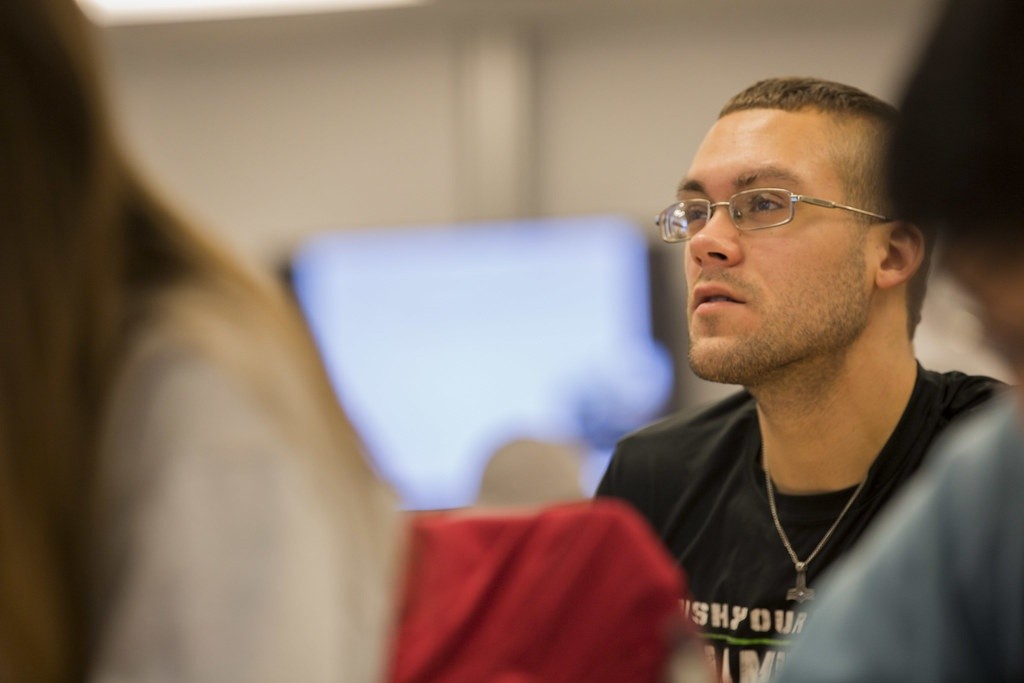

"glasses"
[655,187,893,243]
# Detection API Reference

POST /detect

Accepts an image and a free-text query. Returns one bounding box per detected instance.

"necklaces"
[761,434,872,606]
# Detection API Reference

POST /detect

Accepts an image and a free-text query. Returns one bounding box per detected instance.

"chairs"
[285,212,670,507]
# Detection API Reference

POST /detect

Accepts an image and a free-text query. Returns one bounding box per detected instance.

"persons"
[588,73,1015,683]
[2,1,719,683]
[757,0,1022,682]
